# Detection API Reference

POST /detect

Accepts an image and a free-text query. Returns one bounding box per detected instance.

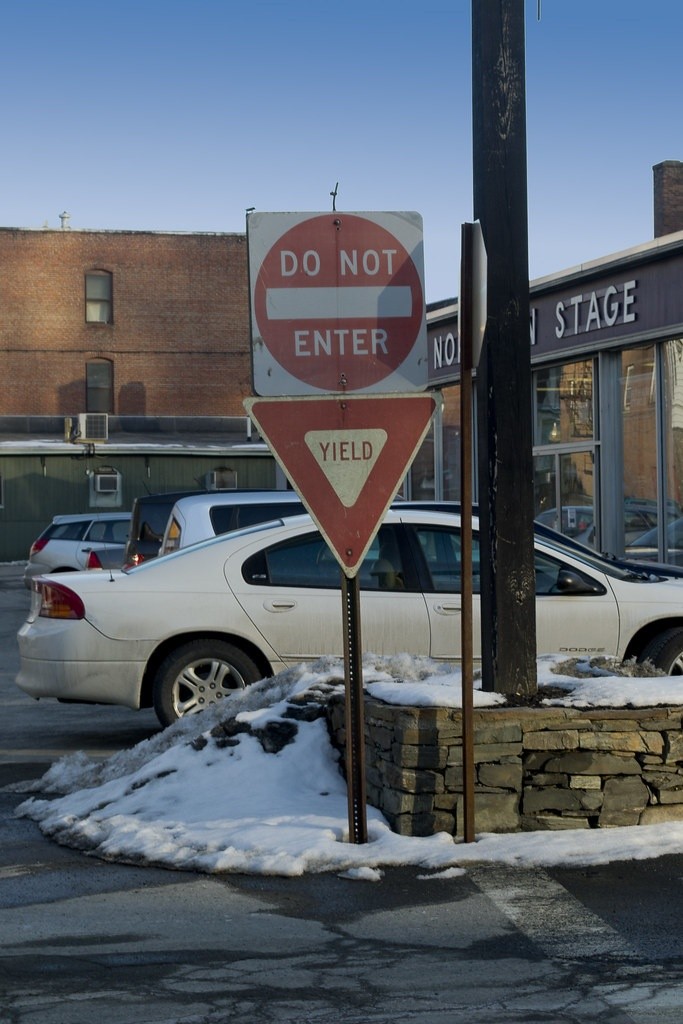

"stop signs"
[250,210,431,397]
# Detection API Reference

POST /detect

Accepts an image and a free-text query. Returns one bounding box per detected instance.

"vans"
[130,496,200,569]
[159,493,683,576]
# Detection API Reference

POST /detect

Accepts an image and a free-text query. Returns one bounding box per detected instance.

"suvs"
[23,513,134,587]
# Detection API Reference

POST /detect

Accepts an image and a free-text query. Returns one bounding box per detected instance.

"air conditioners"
[210,470,238,489]
[77,413,109,442]
[94,473,120,493]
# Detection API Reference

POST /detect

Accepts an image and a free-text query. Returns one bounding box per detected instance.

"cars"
[534,498,683,567]
[15,509,682,730]
[87,548,127,573]
